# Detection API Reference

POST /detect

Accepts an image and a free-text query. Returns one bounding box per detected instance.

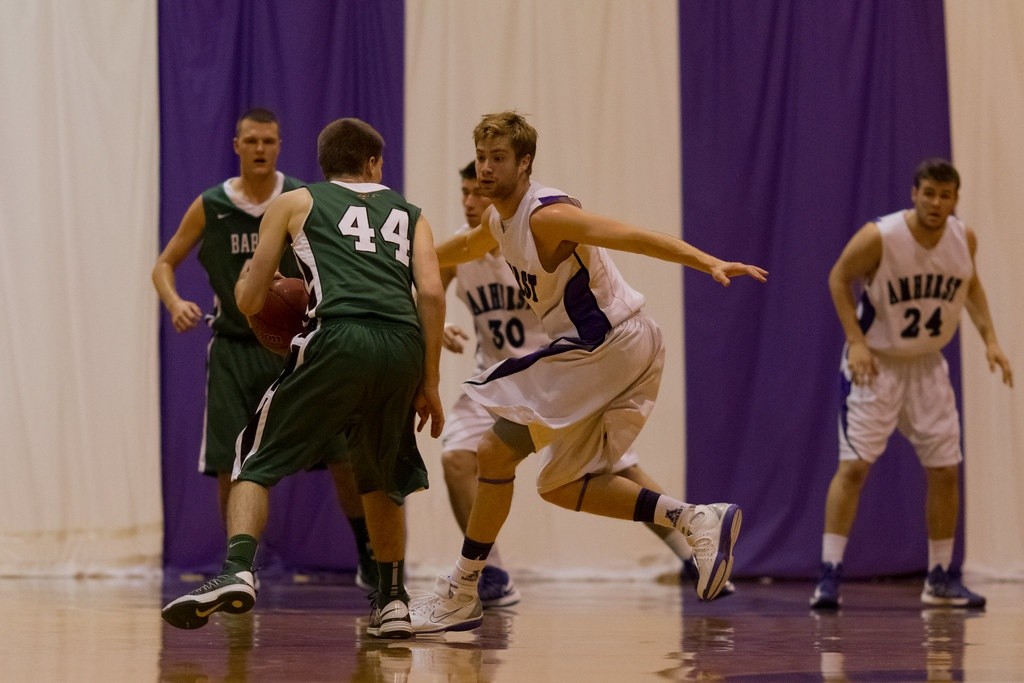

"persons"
[157,118,446,636]
[806,159,1017,615]
[407,110,771,634]
[151,107,413,603]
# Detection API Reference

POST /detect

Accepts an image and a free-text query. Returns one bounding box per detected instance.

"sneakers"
[408,574,484,635]
[366,589,413,638]
[355,560,412,604]
[808,561,844,611]
[921,564,985,609]
[477,564,521,608]
[684,552,735,599]
[679,503,743,600]
[160,566,257,629]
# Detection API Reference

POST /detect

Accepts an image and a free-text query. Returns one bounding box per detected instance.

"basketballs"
[252,275,313,355]
